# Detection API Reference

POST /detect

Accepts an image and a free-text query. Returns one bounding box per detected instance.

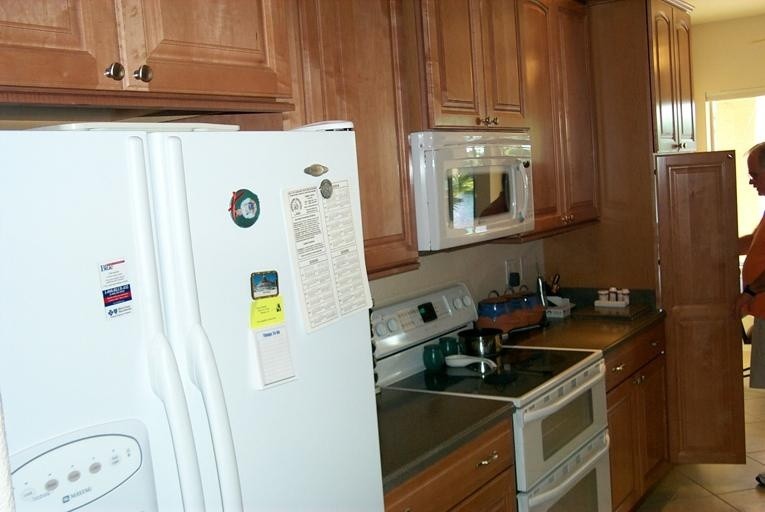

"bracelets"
[743,284,756,297]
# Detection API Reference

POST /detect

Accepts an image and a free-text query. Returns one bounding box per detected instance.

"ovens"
[513,357,612,512]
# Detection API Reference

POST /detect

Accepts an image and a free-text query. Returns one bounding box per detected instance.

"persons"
[736,142,765,487]
[478,172,510,216]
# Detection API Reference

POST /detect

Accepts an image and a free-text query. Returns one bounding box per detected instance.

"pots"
[456,323,543,359]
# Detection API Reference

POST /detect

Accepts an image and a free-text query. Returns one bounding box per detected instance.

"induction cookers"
[386,347,597,398]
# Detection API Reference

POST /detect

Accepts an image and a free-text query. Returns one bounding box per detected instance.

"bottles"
[598,290,609,302]
[422,344,446,373]
[617,291,623,301]
[622,288,631,306]
[609,287,617,301]
[439,336,458,357]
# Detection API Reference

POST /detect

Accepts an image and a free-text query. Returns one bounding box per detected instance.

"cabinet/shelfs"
[492,0,600,243]
[594,1,698,156]
[0,3,295,110]
[383,416,517,512]
[424,0,529,131]
[541,149,748,466]
[288,0,422,281]
[604,321,670,511]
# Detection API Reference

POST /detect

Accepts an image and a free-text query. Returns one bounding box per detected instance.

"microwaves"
[408,127,537,256]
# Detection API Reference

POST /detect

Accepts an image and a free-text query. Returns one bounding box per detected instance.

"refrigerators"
[0,117,385,512]
